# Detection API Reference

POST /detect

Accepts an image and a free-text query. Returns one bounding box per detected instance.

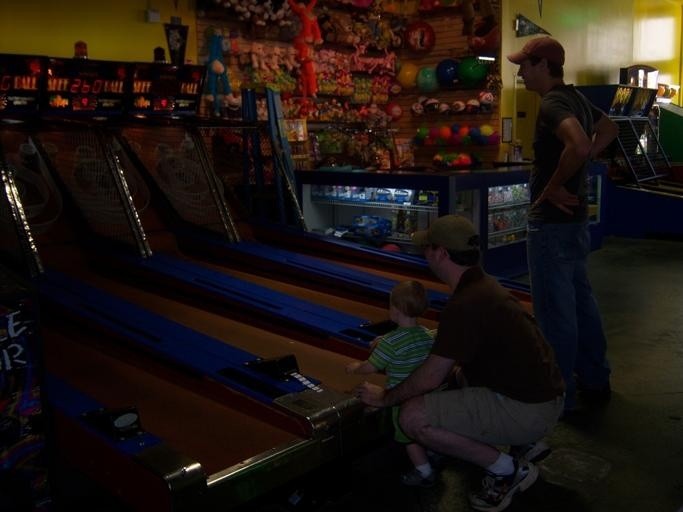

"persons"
[345,281,440,489]
[352,215,567,512]
[508,38,618,412]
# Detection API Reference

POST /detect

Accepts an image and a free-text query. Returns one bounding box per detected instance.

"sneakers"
[498,439,553,472]
[578,377,613,403]
[468,456,541,512]
[399,466,436,488]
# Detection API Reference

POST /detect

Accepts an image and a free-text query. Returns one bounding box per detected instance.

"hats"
[507,36,565,67]
[410,214,482,252]
